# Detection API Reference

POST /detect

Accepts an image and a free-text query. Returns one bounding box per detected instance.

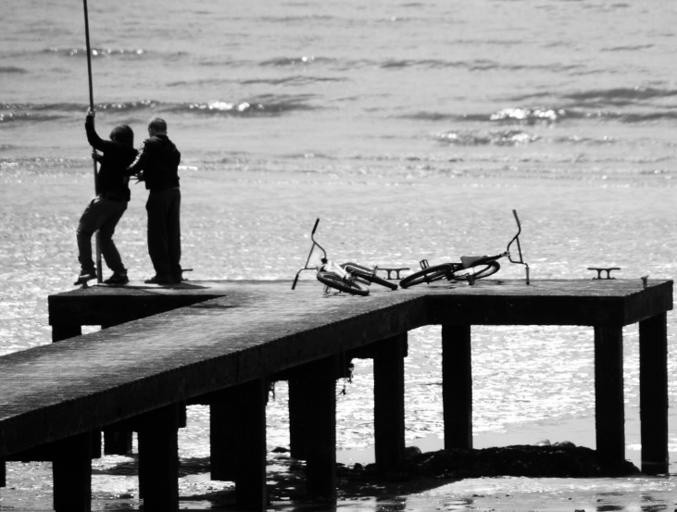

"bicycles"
[396,207,532,290]
[283,215,399,299]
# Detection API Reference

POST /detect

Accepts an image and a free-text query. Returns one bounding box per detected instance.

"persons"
[73,107,139,285]
[122,118,183,285]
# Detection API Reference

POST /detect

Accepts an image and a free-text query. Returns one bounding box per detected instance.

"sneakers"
[74,270,95,285]
[145,273,180,284]
[104,273,128,283]
